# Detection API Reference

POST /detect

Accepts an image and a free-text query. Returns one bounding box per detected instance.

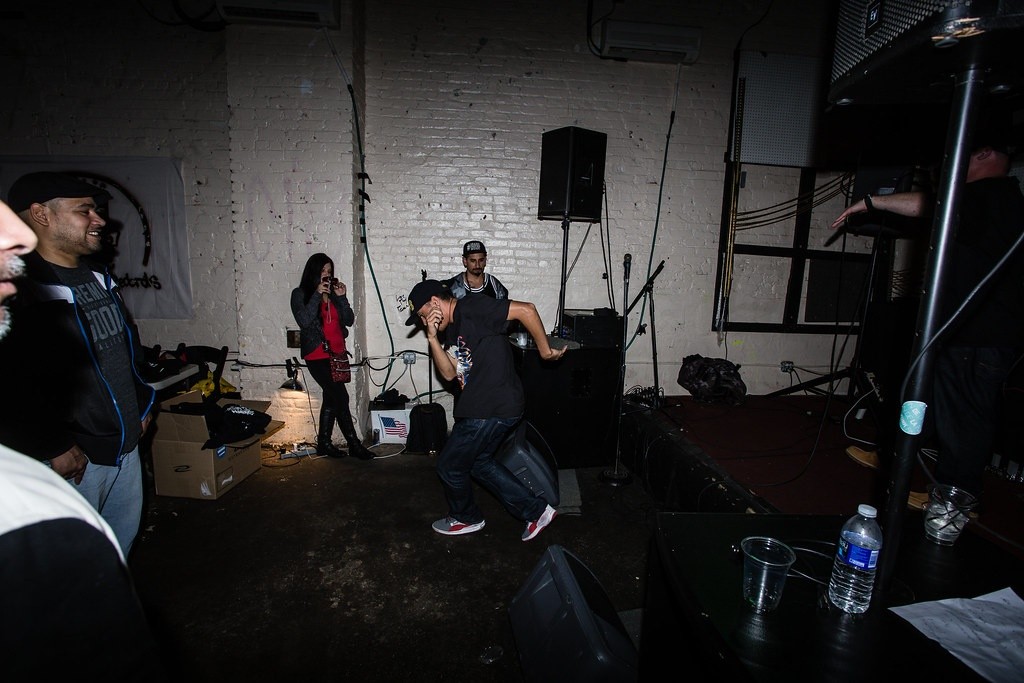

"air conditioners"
[599,18,702,64]
[216,0,340,31]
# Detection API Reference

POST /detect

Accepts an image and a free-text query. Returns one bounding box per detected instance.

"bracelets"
[862,193,874,210]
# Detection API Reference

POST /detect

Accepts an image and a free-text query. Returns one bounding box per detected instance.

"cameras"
[322,276,339,285]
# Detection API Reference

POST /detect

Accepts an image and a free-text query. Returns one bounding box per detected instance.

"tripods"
[759,208,896,456]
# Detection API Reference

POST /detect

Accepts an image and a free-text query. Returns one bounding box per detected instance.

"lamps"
[280,366,305,392]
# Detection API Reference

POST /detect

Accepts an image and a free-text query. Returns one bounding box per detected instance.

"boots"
[337,406,376,460]
[316,405,348,458]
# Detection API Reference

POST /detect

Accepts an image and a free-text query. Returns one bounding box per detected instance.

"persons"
[831,138,1024,477]
[0,199,153,683]
[405,280,569,541]
[439,241,508,410]
[0,170,155,562]
[290,253,376,460]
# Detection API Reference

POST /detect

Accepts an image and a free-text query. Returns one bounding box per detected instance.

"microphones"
[624,254,631,283]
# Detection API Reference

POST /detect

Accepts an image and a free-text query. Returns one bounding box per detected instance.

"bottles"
[830,504,883,612]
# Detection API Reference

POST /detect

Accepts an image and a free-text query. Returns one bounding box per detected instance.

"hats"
[968,121,1023,162]
[405,280,453,326]
[8,171,114,214]
[462,240,486,258]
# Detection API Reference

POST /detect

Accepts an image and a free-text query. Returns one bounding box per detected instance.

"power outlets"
[404,352,416,364]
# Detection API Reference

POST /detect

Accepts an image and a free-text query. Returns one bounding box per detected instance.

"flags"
[378,416,408,438]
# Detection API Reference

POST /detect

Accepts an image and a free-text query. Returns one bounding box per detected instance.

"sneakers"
[433,516,486,535]
[521,503,558,542]
[847,446,879,471]
[906,490,929,510]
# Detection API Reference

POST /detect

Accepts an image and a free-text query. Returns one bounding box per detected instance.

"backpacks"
[406,402,449,454]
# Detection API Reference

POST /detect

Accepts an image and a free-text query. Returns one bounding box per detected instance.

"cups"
[741,536,795,610]
[925,486,977,542]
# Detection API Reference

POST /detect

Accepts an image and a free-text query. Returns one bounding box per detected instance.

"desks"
[624,391,1024,519]
[647,507,1024,683]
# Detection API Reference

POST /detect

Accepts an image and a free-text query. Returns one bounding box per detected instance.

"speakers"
[494,419,560,507]
[824,0,1024,120]
[537,126,607,224]
[508,544,639,683]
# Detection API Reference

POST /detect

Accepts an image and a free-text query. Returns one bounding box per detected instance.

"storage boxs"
[153,389,285,499]
[371,401,417,444]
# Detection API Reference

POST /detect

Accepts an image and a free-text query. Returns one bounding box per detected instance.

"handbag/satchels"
[220,401,272,433]
[329,350,353,383]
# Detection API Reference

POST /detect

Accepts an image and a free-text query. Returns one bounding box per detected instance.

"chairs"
[174,344,229,379]
